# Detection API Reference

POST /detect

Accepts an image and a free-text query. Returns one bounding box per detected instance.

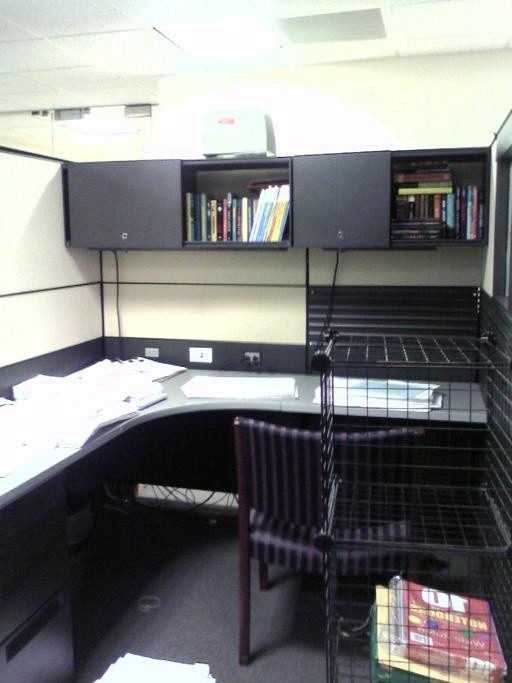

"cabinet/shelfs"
[311,326,512,683]
[63,156,293,251]
[293,146,490,250]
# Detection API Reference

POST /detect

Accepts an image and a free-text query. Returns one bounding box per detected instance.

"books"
[386,158,487,243]
[185,184,292,241]
[369,577,510,683]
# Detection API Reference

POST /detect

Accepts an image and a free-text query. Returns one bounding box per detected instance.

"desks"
[0,368,488,682]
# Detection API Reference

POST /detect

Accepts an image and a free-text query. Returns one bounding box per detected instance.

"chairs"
[234,417,430,666]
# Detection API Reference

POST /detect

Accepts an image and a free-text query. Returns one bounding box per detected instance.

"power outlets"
[244,351,261,371]
